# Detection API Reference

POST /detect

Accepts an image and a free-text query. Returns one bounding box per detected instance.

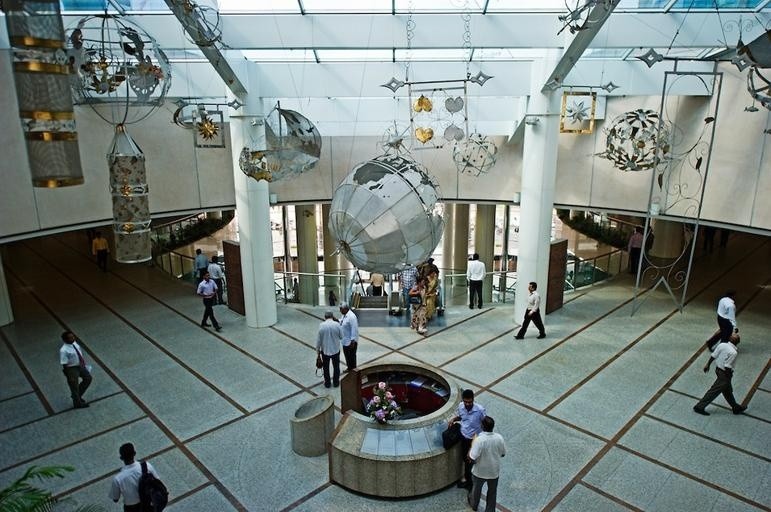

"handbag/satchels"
[441,423,461,450]
[316,356,323,369]
[137,472,168,512]
[408,294,422,304]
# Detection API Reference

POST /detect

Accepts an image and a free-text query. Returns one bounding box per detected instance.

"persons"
[719,228,731,249]
[368,256,443,335]
[449,388,488,490]
[693,332,749,416]
[60,330,93,408]
[641,225,655,272]
[513,282,548,340]
[197,270,224,331]
[206,256,227,304]
[93,230,111,273]
[702,225,718,257]
[192,248,210,294]
[292,277,300,302]
[629,226,645,274]
[467,253,487,310]
[338,300,360,374]
[329,290,339,306]
[316,309,344,389]
[109,442,171,512]
[706,287,739,352]
[467,415,508,511]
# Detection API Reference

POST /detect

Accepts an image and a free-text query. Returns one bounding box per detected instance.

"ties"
[73,344,85,368]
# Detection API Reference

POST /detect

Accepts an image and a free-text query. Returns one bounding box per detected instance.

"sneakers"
[323,369,351,388]
[457,478,474,491]
[514,335,524,341]
[201,322,212,328]
[70,394,90,408]
[694,405,710,416]
[536,334,546,339]
[214,300,228,306]
[469,303,482,310]
[215,326,222,332]
[732,404,748,415]
[706,340,713,352]
